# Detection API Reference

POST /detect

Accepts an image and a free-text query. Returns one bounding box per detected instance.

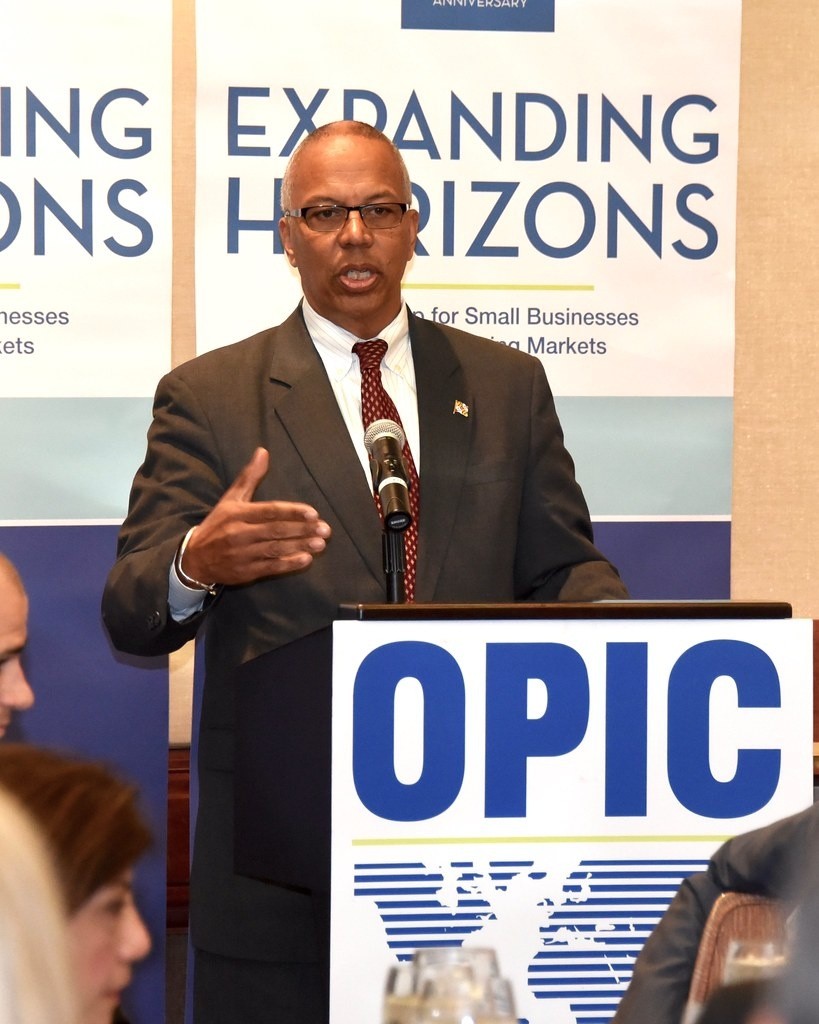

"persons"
[1,550,34,740]
[102,120,634,1023]
[0,744,150,1023]
[610,796,818,1024]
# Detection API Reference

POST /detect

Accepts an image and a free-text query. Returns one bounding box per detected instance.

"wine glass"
[380,944,518,1024]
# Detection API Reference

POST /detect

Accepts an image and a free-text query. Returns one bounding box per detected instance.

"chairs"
[681,884,798,1023]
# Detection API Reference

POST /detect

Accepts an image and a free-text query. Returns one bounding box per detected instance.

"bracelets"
[178,524,219,598]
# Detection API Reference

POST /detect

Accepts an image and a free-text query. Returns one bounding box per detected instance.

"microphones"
[363,418,412,533]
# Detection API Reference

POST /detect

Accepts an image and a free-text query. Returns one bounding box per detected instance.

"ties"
[351,335,425,609]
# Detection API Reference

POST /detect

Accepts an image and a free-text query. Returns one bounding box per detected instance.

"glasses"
[282,197,410,232]
